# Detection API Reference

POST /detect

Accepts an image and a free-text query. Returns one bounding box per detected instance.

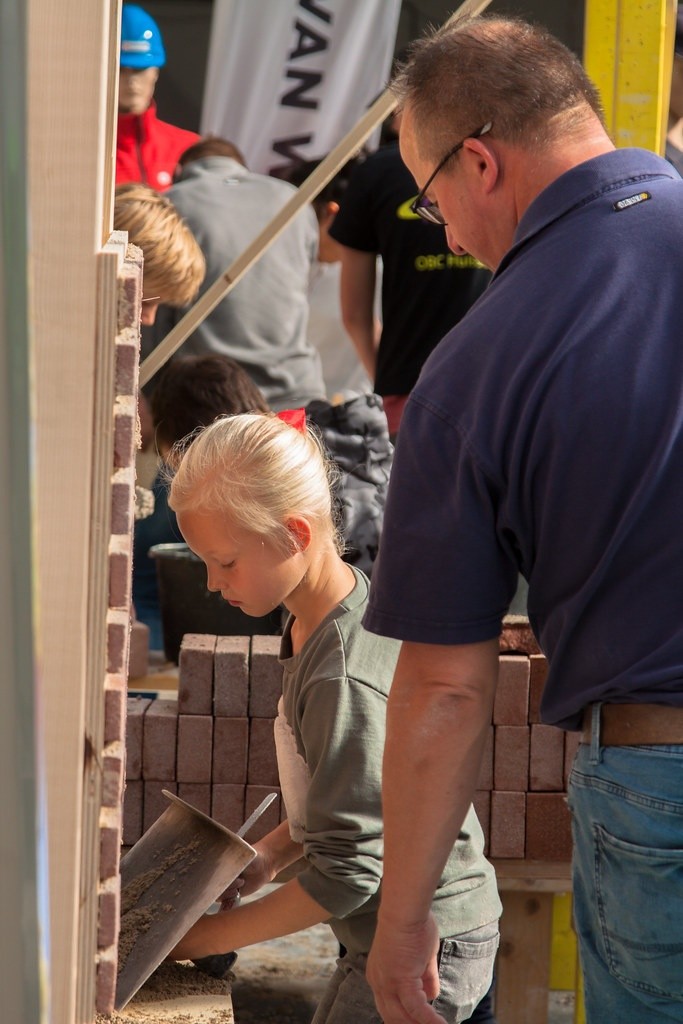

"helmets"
[118,4,164,67]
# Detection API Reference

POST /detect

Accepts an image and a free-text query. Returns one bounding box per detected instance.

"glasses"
[410,122,494,226]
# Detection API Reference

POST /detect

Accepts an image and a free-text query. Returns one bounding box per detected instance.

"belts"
[575,703,682,746]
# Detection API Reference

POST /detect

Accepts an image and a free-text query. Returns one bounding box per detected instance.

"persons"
[113,4,494,1024]
[361,16,683,1024]
